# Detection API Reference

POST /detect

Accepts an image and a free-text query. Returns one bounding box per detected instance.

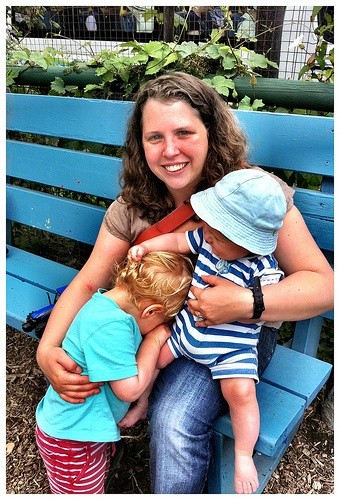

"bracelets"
[249,276,266,320]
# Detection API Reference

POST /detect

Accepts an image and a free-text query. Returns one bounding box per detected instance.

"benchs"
[6,92,334,494]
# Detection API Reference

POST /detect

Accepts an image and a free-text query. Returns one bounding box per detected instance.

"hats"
[190,168,287,256]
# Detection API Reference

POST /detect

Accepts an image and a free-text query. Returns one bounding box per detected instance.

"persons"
[117,166,288,495]
[35,249,194,493]
[34,68,333,495]
